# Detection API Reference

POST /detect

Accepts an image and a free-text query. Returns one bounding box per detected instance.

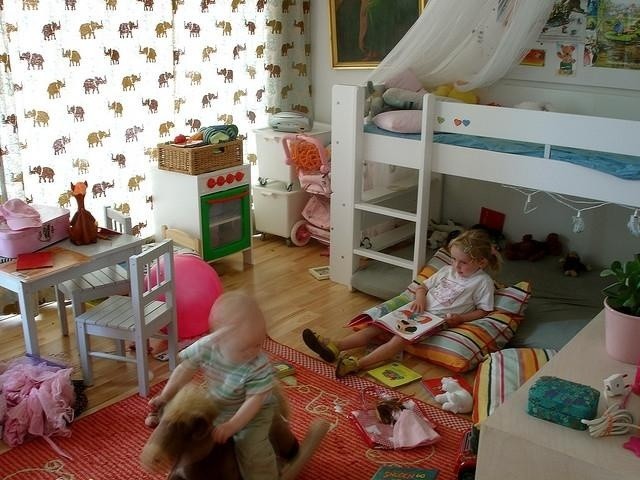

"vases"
[68,181,98,246]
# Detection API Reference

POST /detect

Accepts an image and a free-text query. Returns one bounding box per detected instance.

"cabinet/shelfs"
[153,163,253,266]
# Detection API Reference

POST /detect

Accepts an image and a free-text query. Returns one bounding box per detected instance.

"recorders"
[269,112,313,134]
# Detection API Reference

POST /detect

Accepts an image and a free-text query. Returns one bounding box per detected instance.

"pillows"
[473,346,559,430]
[373,109,444,134]
[354,248,531,373]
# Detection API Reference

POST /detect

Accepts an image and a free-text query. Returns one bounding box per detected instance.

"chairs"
[74,238,178,399]
[53,205,132,338]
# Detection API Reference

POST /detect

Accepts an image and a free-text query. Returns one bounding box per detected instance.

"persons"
[302,229,499,379]
[148,289,280,480]
[376,392,441,450]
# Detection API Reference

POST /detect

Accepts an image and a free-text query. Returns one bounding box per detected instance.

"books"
[367,360,423,388]
[342,295,448,346]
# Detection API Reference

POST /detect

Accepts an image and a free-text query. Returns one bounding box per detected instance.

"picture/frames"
[328,0,430,70]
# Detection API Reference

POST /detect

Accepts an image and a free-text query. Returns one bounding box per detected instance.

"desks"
[0,227,147,356]
[474,308,639,480]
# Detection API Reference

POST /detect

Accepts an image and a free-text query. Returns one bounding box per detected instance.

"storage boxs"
[0,204,72,259]
[252,182,313,247]
[254,122,332,192]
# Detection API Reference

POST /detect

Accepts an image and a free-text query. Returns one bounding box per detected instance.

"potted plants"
[600,251,640,364]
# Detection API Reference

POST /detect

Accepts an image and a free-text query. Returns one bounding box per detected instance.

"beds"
[329,78,640,350]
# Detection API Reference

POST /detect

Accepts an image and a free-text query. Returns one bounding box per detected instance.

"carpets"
[0,334,475,479]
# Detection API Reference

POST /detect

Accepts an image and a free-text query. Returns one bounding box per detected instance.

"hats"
[0,199,43,231]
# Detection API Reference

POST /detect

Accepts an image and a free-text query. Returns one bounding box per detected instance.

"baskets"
[158,138,243,175]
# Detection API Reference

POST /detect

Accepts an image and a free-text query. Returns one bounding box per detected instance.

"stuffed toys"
[434,376,473,414]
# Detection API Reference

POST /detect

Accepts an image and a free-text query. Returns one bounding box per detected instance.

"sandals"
[336,351,360,378]
[302,329,338,363]
